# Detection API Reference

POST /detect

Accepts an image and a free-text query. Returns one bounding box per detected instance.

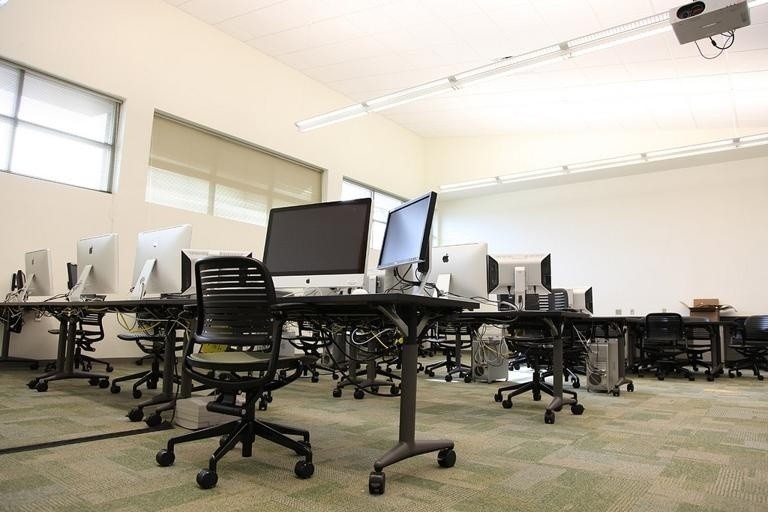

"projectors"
[669,0,752,45]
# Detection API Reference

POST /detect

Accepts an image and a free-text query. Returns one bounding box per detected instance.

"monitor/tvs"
[427,243,488,303]
[573,289,588,314]
[180,249,254,300]
[128,224,193,300]
[262,197,373,295]
[17,270,26,293]
[376,190,437,296]
[65,233,119,302]
[67,263,77,291]
[486,252,551,312]
[17,248,54,302]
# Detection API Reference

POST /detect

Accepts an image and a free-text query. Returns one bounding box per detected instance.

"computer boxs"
[472,336,509,384]
[586,337,624,393]
[321,333,346,369]
